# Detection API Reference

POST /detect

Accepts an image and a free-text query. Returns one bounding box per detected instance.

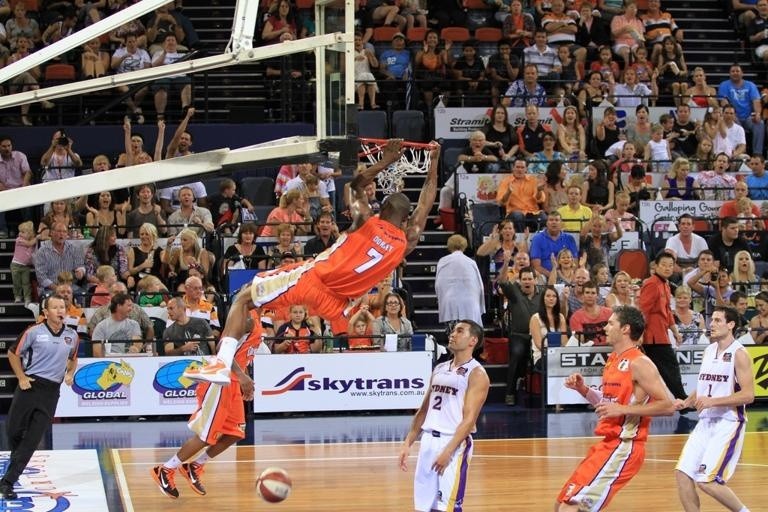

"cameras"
[57,127,69,147]
[710,271,718,281]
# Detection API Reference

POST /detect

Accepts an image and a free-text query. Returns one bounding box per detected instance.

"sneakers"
[183,358,231,384]
[150,463,178,499]
[0,482,18,499]
[178,461,206,495]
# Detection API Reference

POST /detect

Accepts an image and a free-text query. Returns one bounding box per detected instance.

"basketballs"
[256,467,290,502]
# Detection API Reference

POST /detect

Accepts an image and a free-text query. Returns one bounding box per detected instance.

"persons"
[498,251,544,404]
[633,253,696,413]
[182,137,443,386]
[148,281,264,499]
[674,305,754,511]
[1,1,768,353]
[2,294,79,499]
[398,319,490,512]
[550,303,676,511]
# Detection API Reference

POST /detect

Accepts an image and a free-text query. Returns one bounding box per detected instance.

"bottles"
[753,272,761,293]
[146,336,153,352]
[489,259,497,281]
[326,328,334,352]
[69,223,90,240]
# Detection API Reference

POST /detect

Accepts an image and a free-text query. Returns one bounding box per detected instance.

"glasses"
[386,302,400,306]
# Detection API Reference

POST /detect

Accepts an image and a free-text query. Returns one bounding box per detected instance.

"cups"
[104,343,112,354]
